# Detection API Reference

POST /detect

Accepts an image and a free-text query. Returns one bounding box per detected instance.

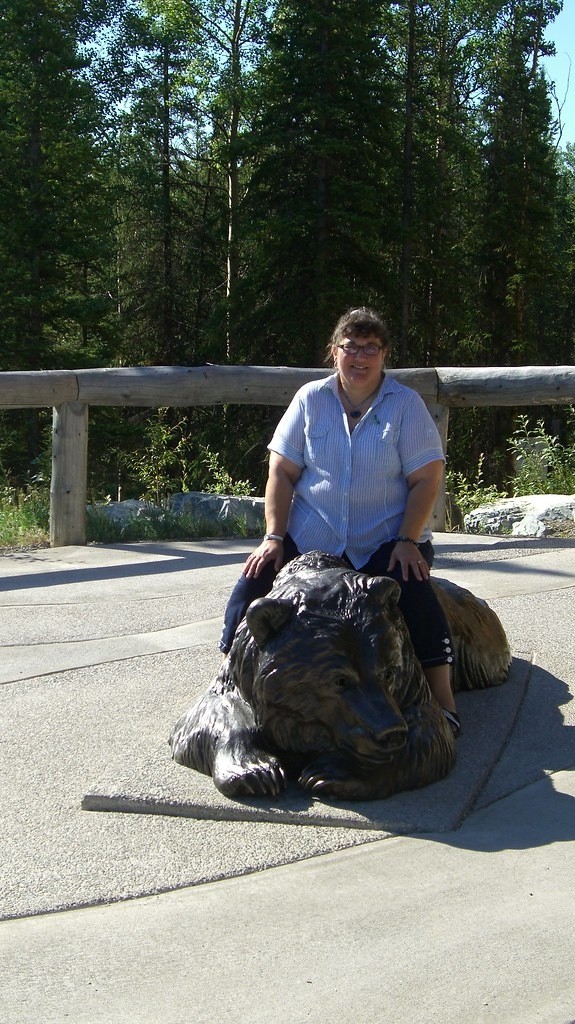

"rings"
[418,559,424,563]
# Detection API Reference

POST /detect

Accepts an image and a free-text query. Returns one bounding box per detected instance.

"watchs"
[262,533,284,544]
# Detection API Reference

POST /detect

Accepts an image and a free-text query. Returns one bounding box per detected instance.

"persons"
[212,308,466,737]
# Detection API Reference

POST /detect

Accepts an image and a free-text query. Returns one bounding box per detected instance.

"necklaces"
[339,379,383,418]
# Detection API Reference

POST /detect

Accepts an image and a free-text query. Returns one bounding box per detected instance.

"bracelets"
[393,534,419,547]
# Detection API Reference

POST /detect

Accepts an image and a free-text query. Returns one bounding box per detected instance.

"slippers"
[442,707,460,737]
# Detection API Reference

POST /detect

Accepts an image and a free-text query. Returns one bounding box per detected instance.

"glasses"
[336,342,388,356]
